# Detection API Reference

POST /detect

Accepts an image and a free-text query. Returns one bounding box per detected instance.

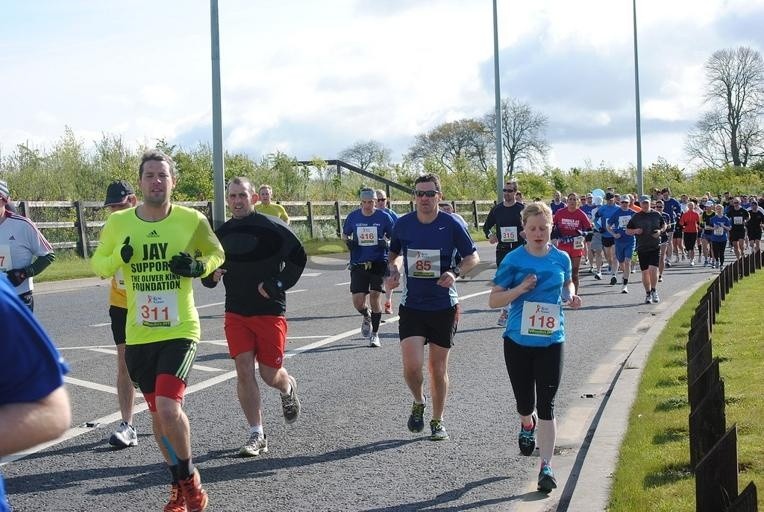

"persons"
[0,179,55,314]
[341,188,396,347]
[441,202,469,266]
[102,178,139,446]
[198,176,308,457]
[254,184,291,225]
[374,189,401,315]
[489,200,582,495]
[0,270,73,511]
[383,171,480,441]
[88,151,225,512]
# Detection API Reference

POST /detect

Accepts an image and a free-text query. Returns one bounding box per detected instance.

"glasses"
[414,189,439,197]
[502,189,515,192]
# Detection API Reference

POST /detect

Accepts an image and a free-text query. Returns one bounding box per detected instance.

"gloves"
[257,279,281,300]
[346,240,357,250]
[168,251,207,278]
[6,268,26,288]
[117,235,134,265]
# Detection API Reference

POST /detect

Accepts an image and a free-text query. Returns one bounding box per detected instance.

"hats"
[104,179,135,208]
[586,189,715,209]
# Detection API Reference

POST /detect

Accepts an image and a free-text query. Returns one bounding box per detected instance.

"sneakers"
[518,412,538,456]
[361,309,372,337]
[369,332,381,347]
[497,308,508,327]
[108,422,139,446]
[163,482,186,511]
[180,468,209,512]
[429,418,449,440]
[408,395,426,433]
[665,247,750,273]
[537,465,557,495]
[239,432,269,457]
[584,260,663,305]
[281,375,301,423]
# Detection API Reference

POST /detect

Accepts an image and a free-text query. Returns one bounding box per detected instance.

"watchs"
[450,267,460,278]
